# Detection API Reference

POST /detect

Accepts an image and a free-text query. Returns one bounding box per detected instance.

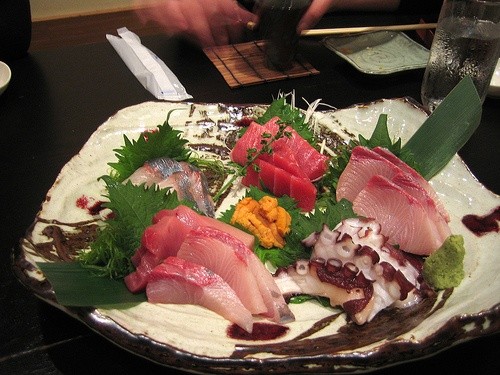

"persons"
[150,0,446,48]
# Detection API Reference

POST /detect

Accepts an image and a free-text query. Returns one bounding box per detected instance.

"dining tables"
[0,13,500,375]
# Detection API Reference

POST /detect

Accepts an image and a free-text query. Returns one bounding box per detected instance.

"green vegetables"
[35,73,483,308]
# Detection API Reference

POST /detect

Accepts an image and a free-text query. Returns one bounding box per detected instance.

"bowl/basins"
[0,60,11,94]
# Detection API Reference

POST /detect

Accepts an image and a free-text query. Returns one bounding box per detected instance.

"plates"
[322,31,452,74]
[8,96,500,375]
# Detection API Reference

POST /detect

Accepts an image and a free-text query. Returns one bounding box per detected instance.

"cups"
[254,0,311,71]
[421,0,500,114]
